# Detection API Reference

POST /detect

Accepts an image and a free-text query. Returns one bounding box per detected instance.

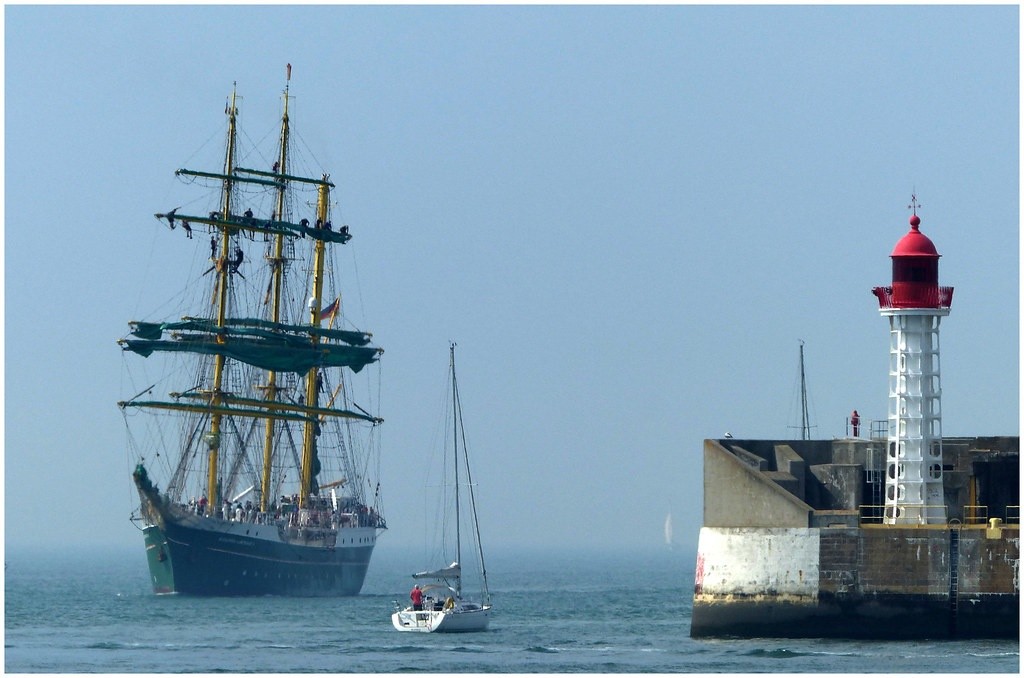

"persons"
[410,584,424,610]
[850,409,861,436]
[183,495,376,527]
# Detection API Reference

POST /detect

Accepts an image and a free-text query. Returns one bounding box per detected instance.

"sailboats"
[114,56,390,598]
[392,339,493,632]
